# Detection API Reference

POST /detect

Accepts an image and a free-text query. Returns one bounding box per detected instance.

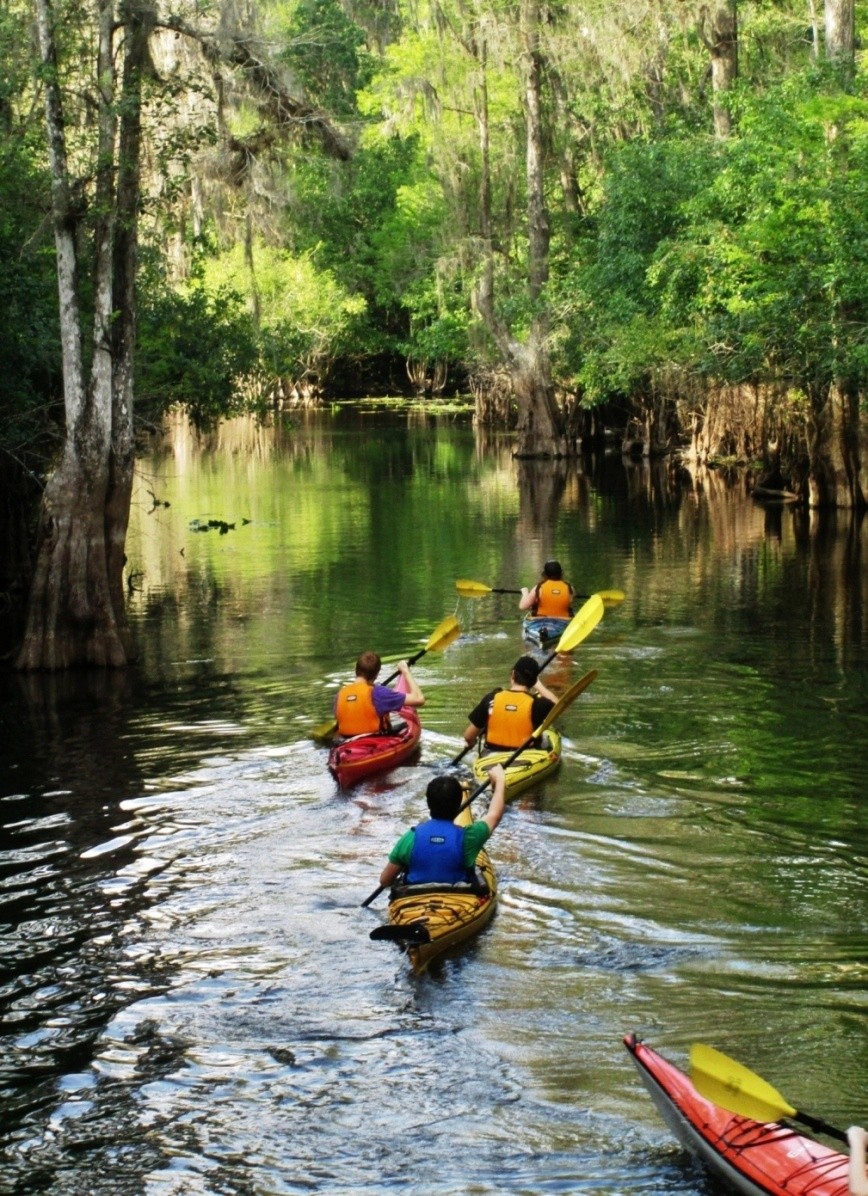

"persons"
[464,656,560,753]
[519,561,576,618]
[379,763,505,887]
[332,651,425,747]
[847,1126,868,1196]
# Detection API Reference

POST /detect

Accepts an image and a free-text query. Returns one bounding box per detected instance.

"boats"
[622,1032,868,1196]
[369,778,500,980]
[522,607,575,644]
[474,723,562,806]
[326,706,422,792]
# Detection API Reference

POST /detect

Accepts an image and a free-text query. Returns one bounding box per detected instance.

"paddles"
[357,668,596,911]
[444,592,604,766]
[690,1039,868,1149]
[305,613,462,743]
[454,578,627,608]
